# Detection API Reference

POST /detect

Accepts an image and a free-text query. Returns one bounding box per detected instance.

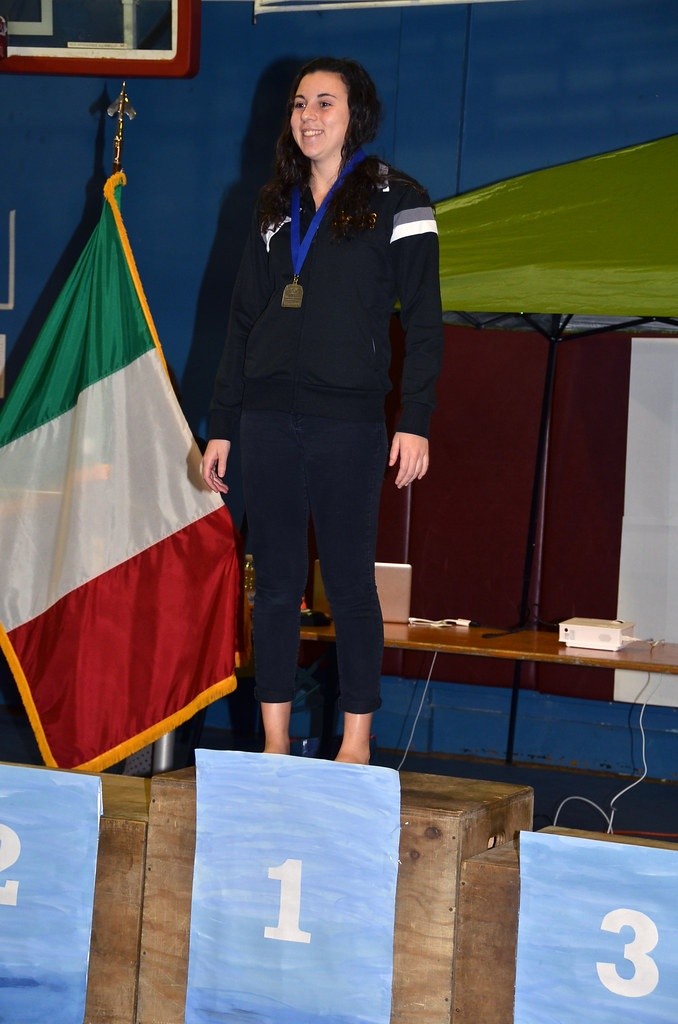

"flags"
[0,169,246,773]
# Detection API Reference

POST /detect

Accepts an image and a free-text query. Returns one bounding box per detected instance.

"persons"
[199,58,446,766]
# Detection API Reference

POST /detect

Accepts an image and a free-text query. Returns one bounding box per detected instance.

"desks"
[247,610,678,765]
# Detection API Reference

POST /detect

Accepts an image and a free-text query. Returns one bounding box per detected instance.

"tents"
[387,131,678,770]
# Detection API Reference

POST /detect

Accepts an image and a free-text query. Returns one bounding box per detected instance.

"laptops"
[312,559,412,624]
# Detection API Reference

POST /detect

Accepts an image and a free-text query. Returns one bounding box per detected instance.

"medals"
[281,284,304,308]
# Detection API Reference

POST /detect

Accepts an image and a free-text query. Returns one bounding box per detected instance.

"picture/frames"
[0,0,201,78]
[254,0,511,17]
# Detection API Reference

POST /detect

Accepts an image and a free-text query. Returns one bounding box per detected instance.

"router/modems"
[558,616,636,653]
